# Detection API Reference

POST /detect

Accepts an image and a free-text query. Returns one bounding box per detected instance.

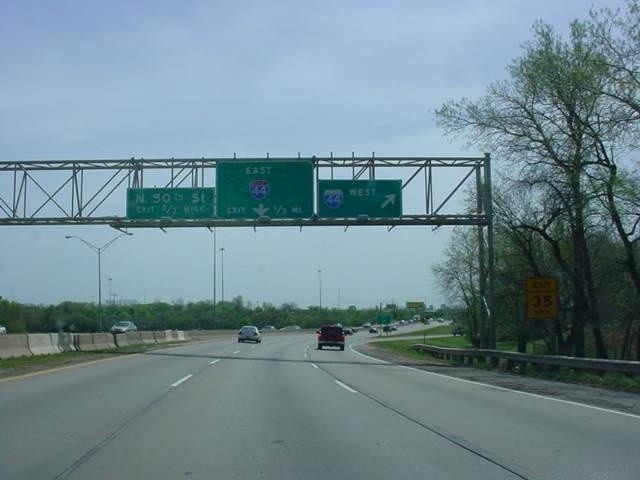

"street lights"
[65,233,133,333]
[108,278,112,304]
[220,248,225,304]
[318,270,322,310]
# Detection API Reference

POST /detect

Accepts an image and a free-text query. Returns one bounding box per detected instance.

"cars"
[452,327,464,336]
[110,321,137,333]
[263,326,300,331]
[369,315,444,333]
[316,324,357,351]
[0,325,6,334]
[238,326,262,343]
[363,322,371,327]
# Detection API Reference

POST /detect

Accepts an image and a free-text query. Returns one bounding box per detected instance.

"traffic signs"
[526,278,559,319]
[216,159,402,219]
